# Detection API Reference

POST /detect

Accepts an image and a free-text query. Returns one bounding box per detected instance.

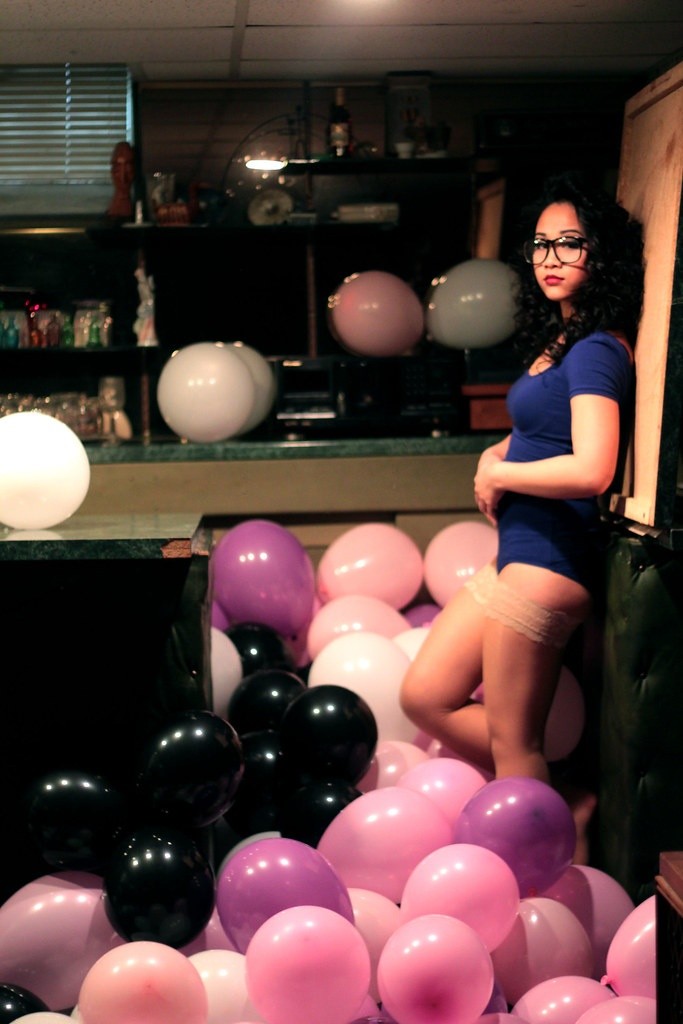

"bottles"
[0,302,112,347]
[330,87,352,159]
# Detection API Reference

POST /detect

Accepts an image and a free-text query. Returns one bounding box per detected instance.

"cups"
[0,392,98,436]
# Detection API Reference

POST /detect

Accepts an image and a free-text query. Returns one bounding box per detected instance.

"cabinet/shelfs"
[0,554,207,903]
[0,166,464,440]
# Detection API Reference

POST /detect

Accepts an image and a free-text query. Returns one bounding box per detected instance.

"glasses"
[523,236,589,265]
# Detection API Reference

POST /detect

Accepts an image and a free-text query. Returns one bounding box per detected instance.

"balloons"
[423,520,498,608]
[425,257,524,349]
[317,523,422,611]
[0,592,656,1023]
[212,520,316,642]
[0,411,91,530]
[157,342,274,445]
[333,270,423,357]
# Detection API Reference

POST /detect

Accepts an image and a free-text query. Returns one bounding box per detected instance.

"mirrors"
[0,80,627,464]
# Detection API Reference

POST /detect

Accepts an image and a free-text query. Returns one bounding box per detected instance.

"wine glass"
[99,376,124,448]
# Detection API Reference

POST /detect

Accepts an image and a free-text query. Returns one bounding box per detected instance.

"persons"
[400,190,646,787]
